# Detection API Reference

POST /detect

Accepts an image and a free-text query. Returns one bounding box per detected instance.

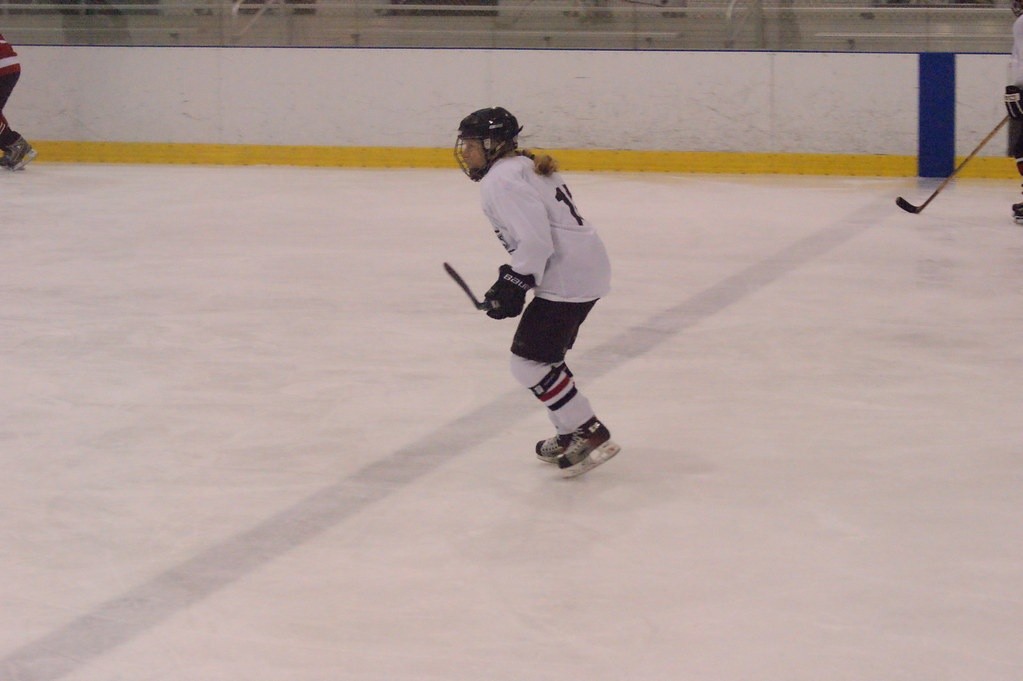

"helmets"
[454,107,523,182]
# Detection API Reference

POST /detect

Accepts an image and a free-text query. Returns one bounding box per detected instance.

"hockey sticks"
[896,112,1010,215]
[441,260,503,311]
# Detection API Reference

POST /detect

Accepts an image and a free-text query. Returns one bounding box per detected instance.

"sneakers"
[535,434,573,464]
[1012,201,1023,219]
[0,132,37,171]
[558,416,620,478]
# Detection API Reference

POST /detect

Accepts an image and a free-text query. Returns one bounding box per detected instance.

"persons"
[1005,0,1023,224]
[454,107,620,480]
[0,33,37,171]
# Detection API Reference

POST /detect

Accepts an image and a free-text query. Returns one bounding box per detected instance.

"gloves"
[485,265,535,320]
[1004,86,1023,119]
